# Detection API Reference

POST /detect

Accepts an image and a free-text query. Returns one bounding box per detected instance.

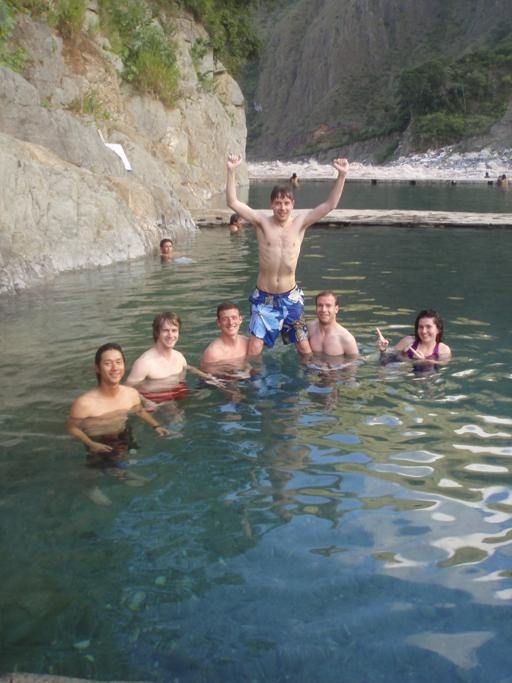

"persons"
[64,343,169,508]
[498,176,508,188]
[159,239,175,259]
[225,154,350,356]
[202,303,250,401]
[289,173,298,187]
[376,309,452,371]
[305,291,358,356]
[226,212,242,234]
[124,313,224,409]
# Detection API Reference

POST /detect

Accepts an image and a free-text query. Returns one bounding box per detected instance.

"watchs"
[151,424,159,428]
